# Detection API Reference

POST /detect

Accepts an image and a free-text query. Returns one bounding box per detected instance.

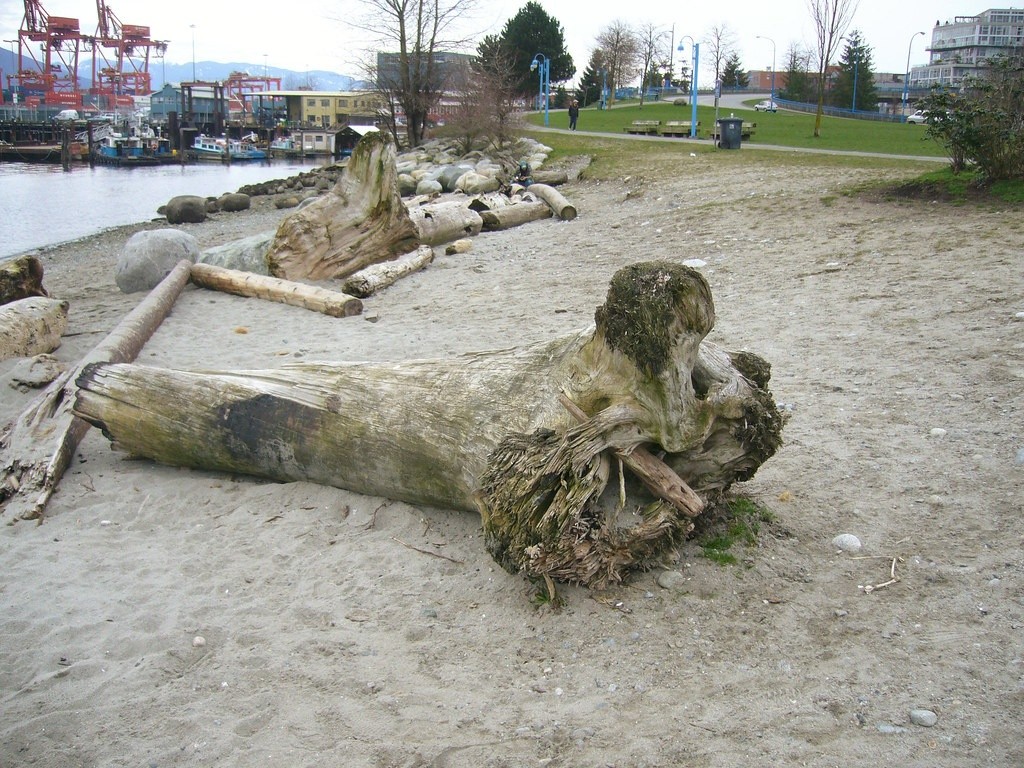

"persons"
[568,99,579,132]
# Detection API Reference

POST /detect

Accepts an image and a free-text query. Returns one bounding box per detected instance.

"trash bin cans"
[719,117,743,149]
[597,100,603,109]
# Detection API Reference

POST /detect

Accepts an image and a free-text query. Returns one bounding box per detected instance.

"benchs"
[661,121,701,138]
[622,120,661,136]
[705,122,758,141]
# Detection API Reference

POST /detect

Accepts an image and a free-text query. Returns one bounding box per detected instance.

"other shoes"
[573,129,576,131]
[568,127,571,130]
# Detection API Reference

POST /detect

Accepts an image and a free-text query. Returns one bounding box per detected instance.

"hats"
[573,99,579,102]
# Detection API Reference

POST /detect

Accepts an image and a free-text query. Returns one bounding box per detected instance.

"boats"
[190,133,266,159]
[270,137,302,160]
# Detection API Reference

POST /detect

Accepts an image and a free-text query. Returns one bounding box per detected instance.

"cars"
[755,101,778,113]
[906,110,957,125]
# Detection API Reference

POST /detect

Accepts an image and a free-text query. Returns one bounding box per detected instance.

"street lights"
[898,32,926,122]
[191,24,197,82]
[530,63,545,113]
[596,67,607,111]
[840,36,859,113]
[532,54,550,126]
[678,35,698,140]
[756,36,774,114]
